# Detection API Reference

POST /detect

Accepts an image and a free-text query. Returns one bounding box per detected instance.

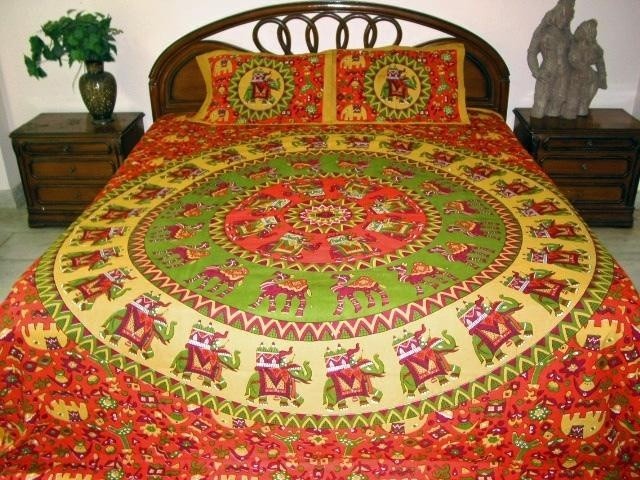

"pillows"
[189,49,333,126]
[331,43,471,125]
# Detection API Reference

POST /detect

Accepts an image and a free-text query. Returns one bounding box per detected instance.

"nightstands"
[9,112,145,228]
[513,107,640,229]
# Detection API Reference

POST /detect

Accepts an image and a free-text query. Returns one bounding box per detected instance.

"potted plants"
[23,8,124,125]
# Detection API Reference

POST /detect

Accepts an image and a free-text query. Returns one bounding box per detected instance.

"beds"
[0,0,640,480]
